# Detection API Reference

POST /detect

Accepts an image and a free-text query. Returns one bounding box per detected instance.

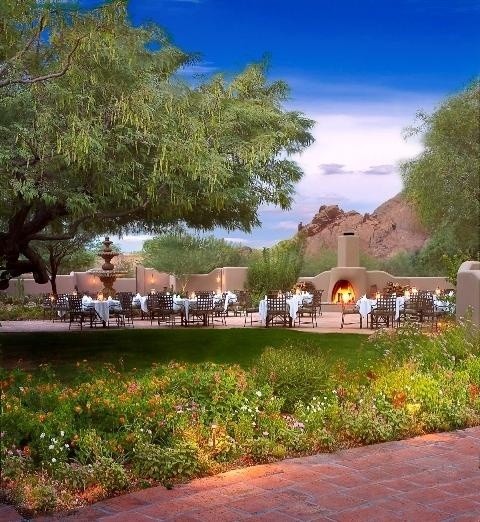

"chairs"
[340,288,457,332]
[40,289,324,331]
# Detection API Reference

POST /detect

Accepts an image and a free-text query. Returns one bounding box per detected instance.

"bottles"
[278,289,282,298]
[97,290,104,301]
[435,286,441,296]
[72,288,77,298]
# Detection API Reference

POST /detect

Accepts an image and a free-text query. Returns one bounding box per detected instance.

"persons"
[81,290,93,311]
